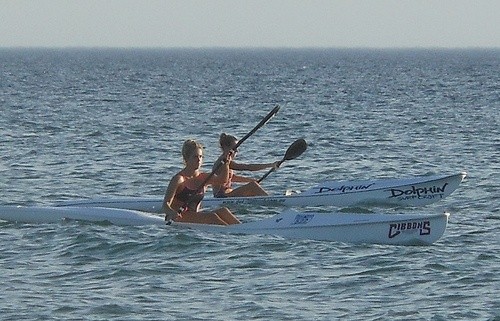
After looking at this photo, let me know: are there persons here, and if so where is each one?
[162,139,243,226]
[212,132,280,198]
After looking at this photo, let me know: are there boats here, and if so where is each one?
[0,204,451,247]
[52,171,468,213]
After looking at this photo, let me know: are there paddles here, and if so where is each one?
[257,139,308,183]
[165,105,281,225]
